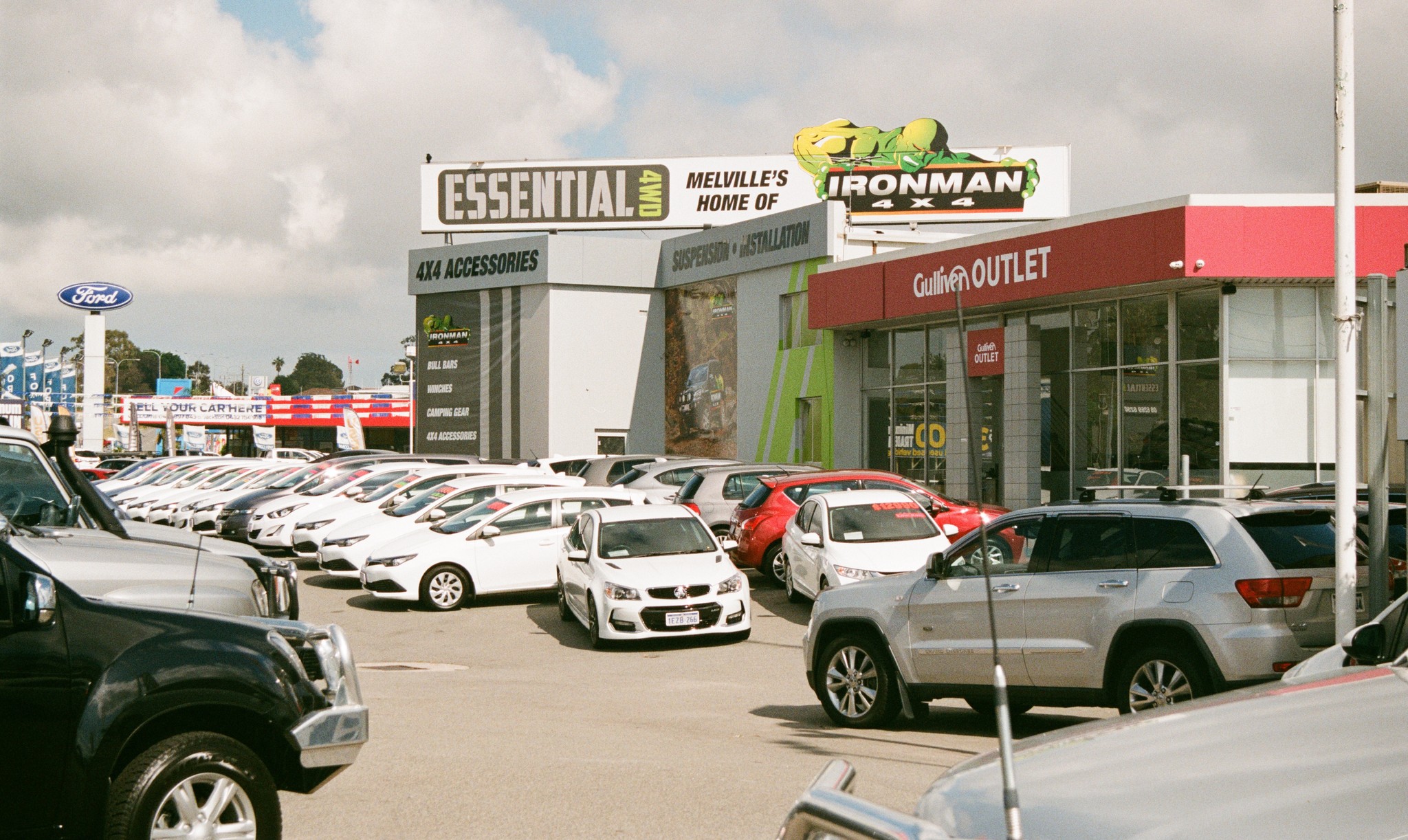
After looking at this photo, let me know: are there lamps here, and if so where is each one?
[703,223,712,231]
[549,228,558,235]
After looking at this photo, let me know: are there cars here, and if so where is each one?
[1280,592,1408,681]
[556,504,751,648]
[317,471,586,578]
[359,484,653,611]
[782,481,966,603]
[906,450,1221,511]
[50,448,480,544]
[290,462,544,558]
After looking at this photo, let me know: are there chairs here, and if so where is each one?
[536,502,565,525]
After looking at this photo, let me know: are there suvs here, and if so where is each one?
[1292,499,1407,601]
[0,511,367,840]
[677,359,725,436]
[1263,479,1407,503]
[672,464,827,567]
[575,456,700,488]
[803,473,1396,729]
[725,465,1025,589]
[772,277,1408,840]
[536,455,607,475]
[0,415,299,623]
[606,458,747,505]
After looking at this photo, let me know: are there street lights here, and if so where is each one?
[105,357,141,413]
[60,346,71,406]
[393,346,416,454]
[41,339,54,410]
[174,350,251,397]
[74,354,85,417]
[22,329,34,399]
[75,361,115,366]
[144,350,173,378]
